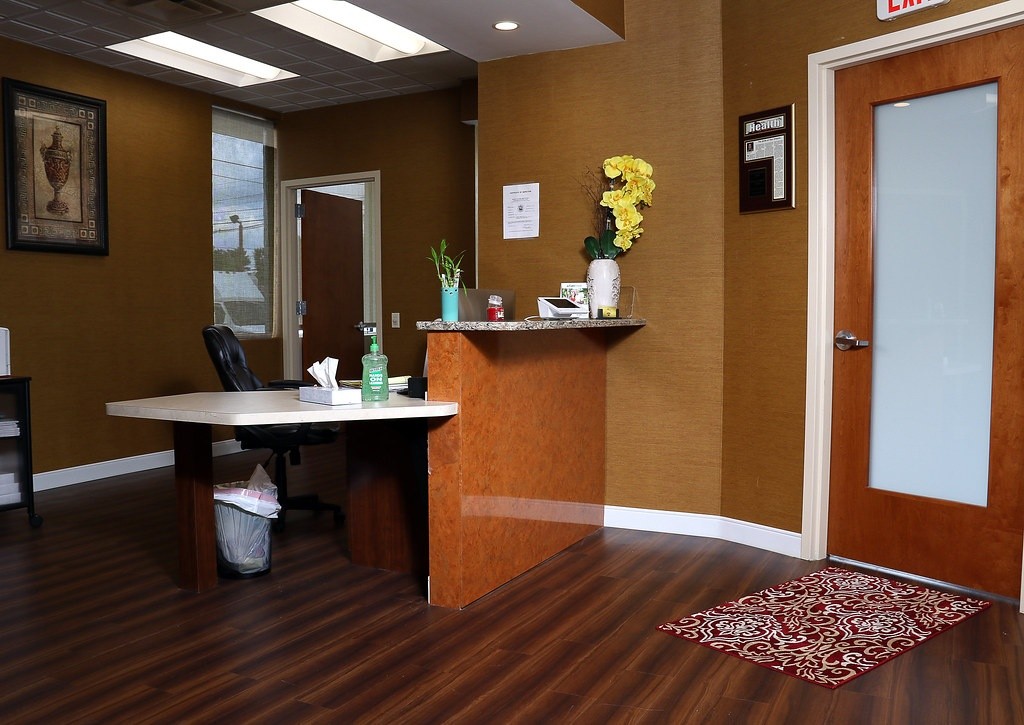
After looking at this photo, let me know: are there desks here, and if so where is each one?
[105,391,457,596]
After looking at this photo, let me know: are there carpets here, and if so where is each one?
[655,566,992,690]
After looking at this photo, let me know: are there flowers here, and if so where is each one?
[573,155,657,259]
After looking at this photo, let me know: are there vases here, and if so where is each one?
[586,259,621,319]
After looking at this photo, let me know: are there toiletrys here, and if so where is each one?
[362,336,389,401]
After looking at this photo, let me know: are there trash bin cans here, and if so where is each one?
[213,477,282,576]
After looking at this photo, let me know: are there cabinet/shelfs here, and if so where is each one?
[0,374,43,528]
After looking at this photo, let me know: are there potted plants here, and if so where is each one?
[426,240,467,322]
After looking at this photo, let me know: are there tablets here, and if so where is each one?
[538,297,589,314]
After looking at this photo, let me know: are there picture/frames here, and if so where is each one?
[0,77,109,257]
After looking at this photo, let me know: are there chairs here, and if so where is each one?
[201,323,345,535]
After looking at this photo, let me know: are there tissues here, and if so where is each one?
[299,358,361,405]
